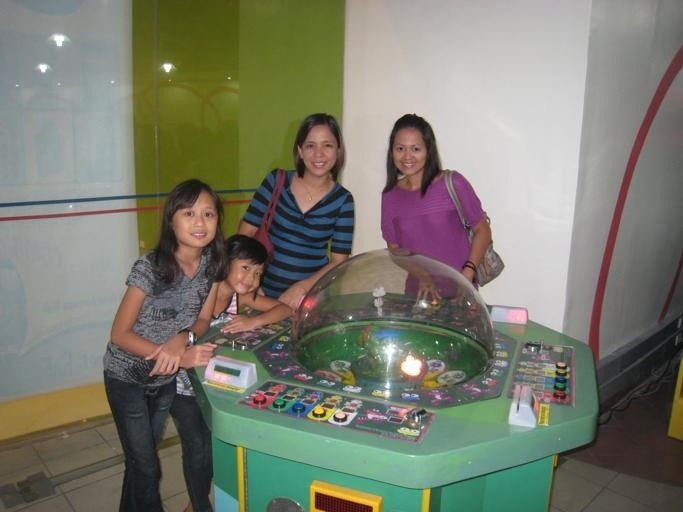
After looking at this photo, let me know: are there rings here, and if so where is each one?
[459,294,466,299]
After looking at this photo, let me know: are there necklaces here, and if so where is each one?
[300,176,329,201]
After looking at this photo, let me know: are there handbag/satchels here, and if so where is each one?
[256,223,273,265]
[465,227,504,287]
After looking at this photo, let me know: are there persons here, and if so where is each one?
[378,111,492,308]
[169,233,294,511]
[100,176,230,511]
[235,112,356,315]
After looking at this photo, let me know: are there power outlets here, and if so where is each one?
[675,318,682,347]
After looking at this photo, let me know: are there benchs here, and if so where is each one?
[0,382,114,448]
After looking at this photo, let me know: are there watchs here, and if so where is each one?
[176,327,197,350]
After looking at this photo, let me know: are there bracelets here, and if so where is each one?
[463,260,476,269]
[462,264,478,274]
